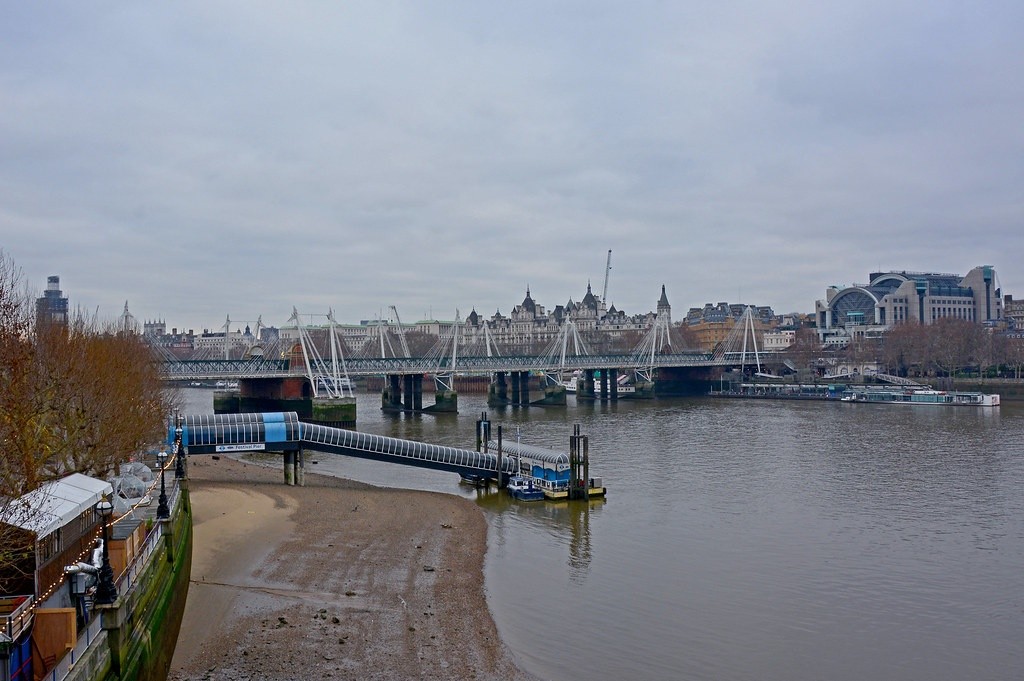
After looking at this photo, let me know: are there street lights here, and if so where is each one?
[157,445,170,518]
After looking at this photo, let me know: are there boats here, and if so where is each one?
[216,381,238,388]
[708,374,829,400]
[840,383,1001,407]
[481,425,572,502]
[558,370,635,395]
